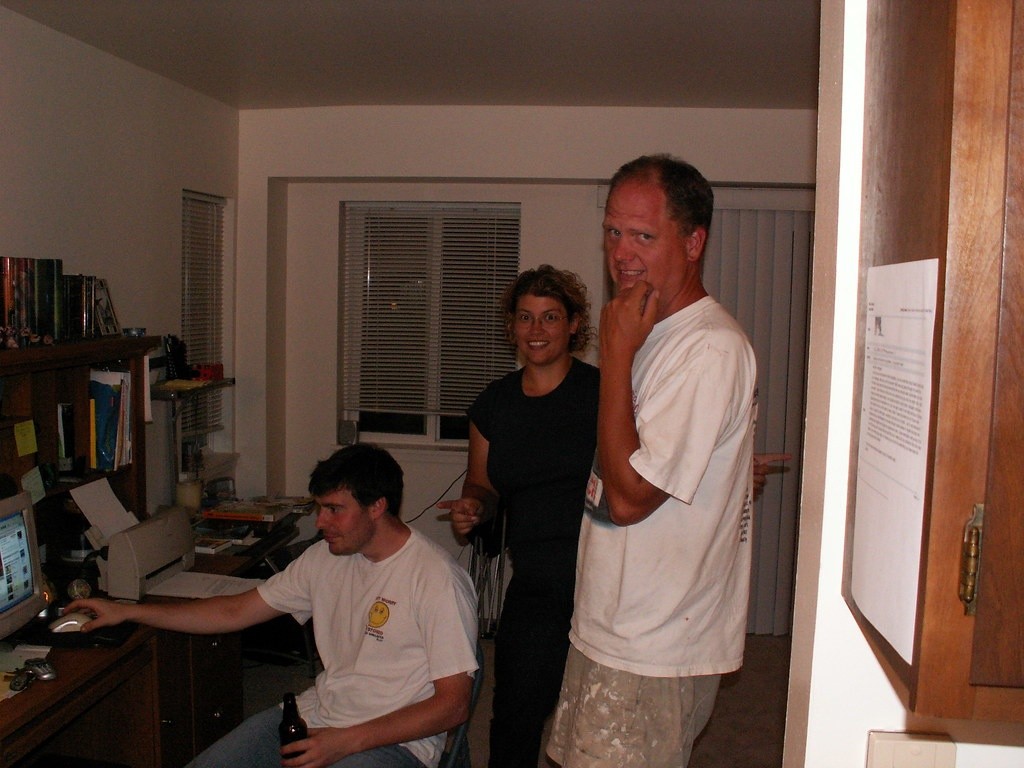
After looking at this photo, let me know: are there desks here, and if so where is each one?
[160,491,318,768]
[1,597,167,768]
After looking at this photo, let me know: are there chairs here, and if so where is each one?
[439,638,484,768]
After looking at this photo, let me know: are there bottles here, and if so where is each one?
[279,692,307,760]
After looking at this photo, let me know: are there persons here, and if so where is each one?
[547,155,756,768]
[63,444,479,768]
[437,265,792,768]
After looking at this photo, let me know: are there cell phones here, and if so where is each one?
[25,658,57,681]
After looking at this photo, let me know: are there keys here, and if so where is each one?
[3,663,37,692]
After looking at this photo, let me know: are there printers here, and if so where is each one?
[83,503,195,601]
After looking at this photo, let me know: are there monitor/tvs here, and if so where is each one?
[0,490,44,640]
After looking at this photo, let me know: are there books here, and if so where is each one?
[90,371,132,473]
[0,257,133,350]
[195,497,315,555]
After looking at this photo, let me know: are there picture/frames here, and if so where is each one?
[91,279,121,336]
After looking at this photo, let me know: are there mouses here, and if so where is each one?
[48,612,92,632]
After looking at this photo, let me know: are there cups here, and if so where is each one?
[176,484,201,516]
[61,559,98,602]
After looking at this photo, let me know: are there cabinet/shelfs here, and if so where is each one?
[158,376,238,488]
[1,337,161,596]
